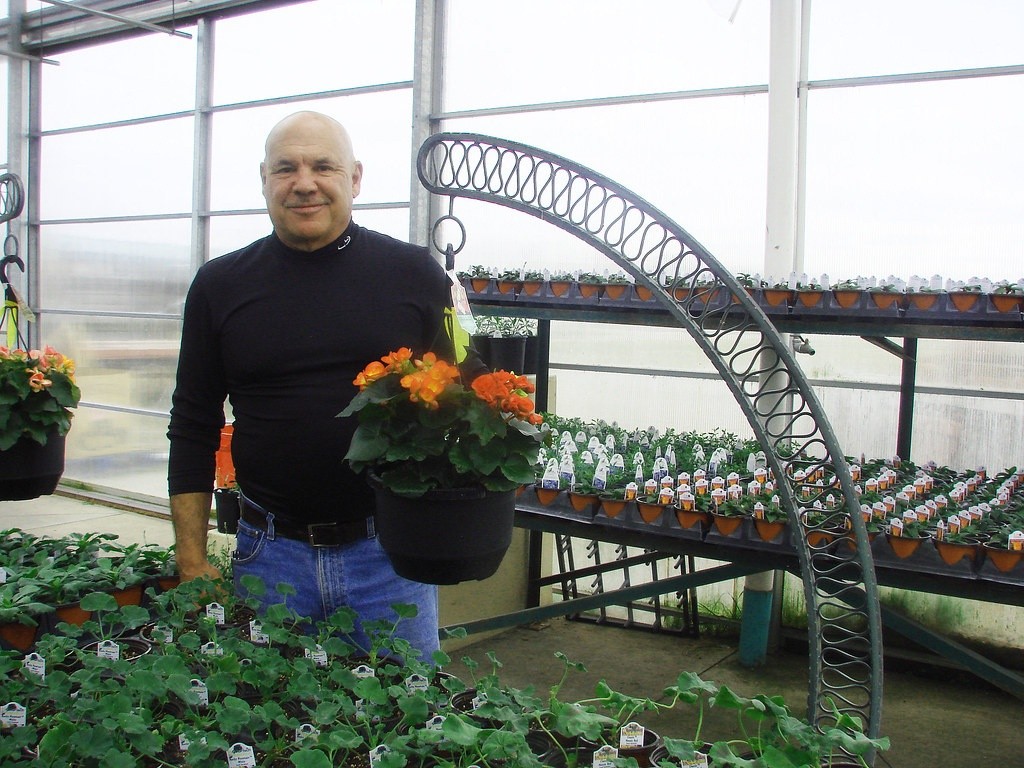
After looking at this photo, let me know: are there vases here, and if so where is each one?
[0,434,64,501]
[372,479,515,587]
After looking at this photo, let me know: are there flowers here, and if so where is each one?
[0,342,81,451]
[333,345,555,500]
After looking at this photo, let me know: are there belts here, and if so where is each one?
[237,505,377,548]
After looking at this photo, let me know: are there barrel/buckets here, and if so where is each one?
[215,425,236,489]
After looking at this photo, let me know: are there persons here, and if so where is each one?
[166,110,491,673]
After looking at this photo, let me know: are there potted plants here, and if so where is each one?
[0,261,1024,768]
